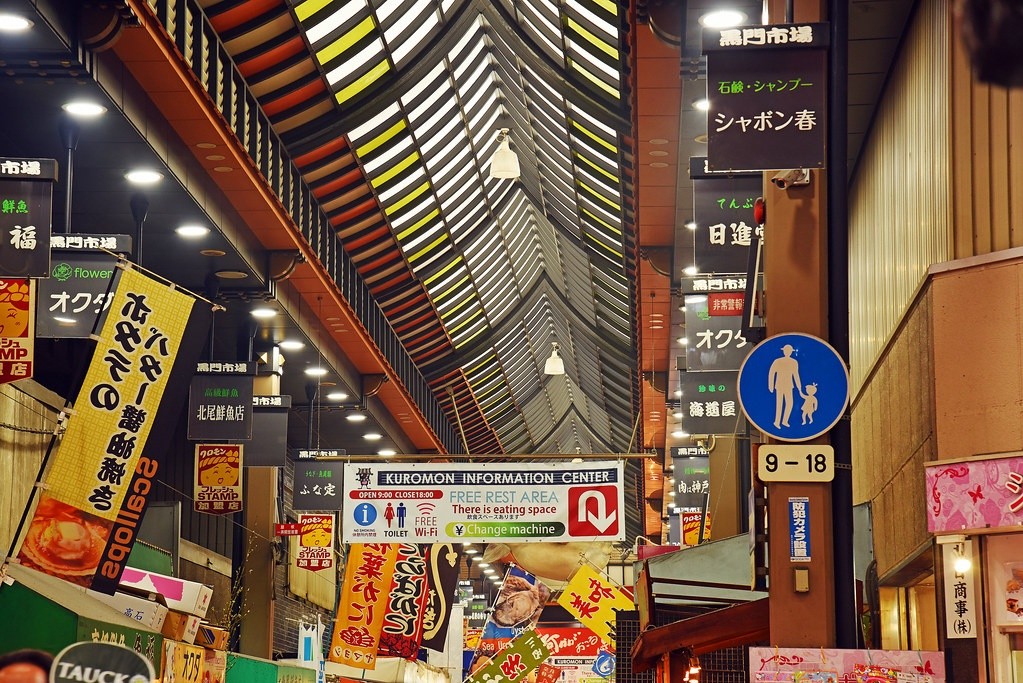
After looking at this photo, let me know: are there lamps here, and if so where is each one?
[544,342,564,375]
[489,128,521,179]
[571,448,582,462]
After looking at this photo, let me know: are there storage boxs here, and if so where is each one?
[84,564,229,650]
